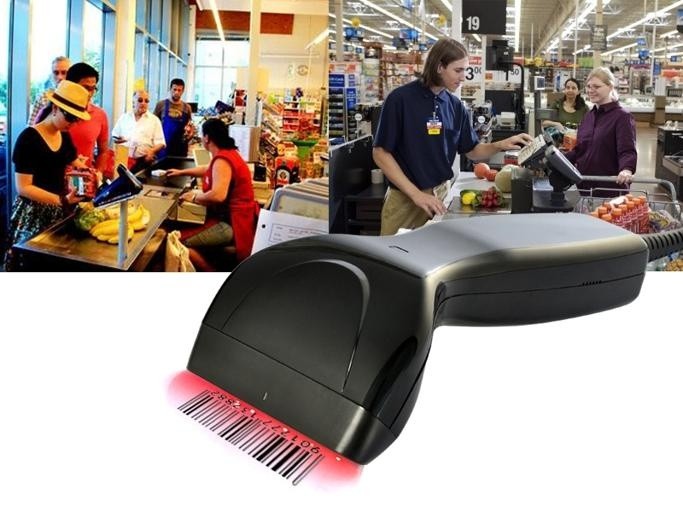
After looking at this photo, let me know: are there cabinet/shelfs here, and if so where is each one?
[580,96,683,122]
[260,94,329,178]
[329,38,418,144]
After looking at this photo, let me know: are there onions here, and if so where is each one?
[473,162,490,179]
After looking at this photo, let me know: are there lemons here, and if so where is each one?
[461,191,476,205]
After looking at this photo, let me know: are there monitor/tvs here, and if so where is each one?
[486,46,514,73]
[540,141,584,194]
[194,147,211,187]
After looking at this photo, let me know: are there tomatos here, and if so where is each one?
[487,170,498,182]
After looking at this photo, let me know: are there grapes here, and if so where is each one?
[481,186,501,207]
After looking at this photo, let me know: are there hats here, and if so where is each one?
[46,80,91,121]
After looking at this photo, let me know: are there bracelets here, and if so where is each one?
[59,193,71,208]
[192,195,197,203]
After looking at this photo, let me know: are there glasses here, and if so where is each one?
[137,98,150,103]
[59,108,79,123]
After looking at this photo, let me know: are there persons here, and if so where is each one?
[562,66,638,199]
[1,78,97,272]
[541,76,589,135]
[111,89,167,170]
[370,37,535,236]
[33,61,110,193]
[152,76,195,159]
[25,55,71,125]
[163,117,256,270]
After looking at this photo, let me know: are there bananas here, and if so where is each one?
[103,198,151,230]
[89,218,134,243]
